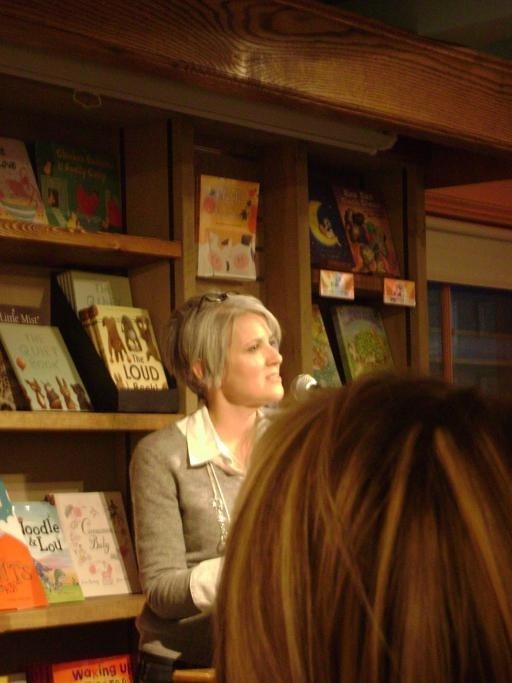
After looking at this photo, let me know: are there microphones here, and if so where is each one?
[292,373,319,403]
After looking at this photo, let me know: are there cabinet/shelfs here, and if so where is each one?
[1,46,429,633]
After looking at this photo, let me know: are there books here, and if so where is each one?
[0,137,125,237]
[0,262,169,412]
[309,181,403,279]
[0,478,147,613]
[1,652,158,683]
[311,301,399,383]
[195,173,261,283]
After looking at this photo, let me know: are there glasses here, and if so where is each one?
[192,290,241,313]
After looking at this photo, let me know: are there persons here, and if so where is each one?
[211,366,511,683]
[128,291,286,683]
